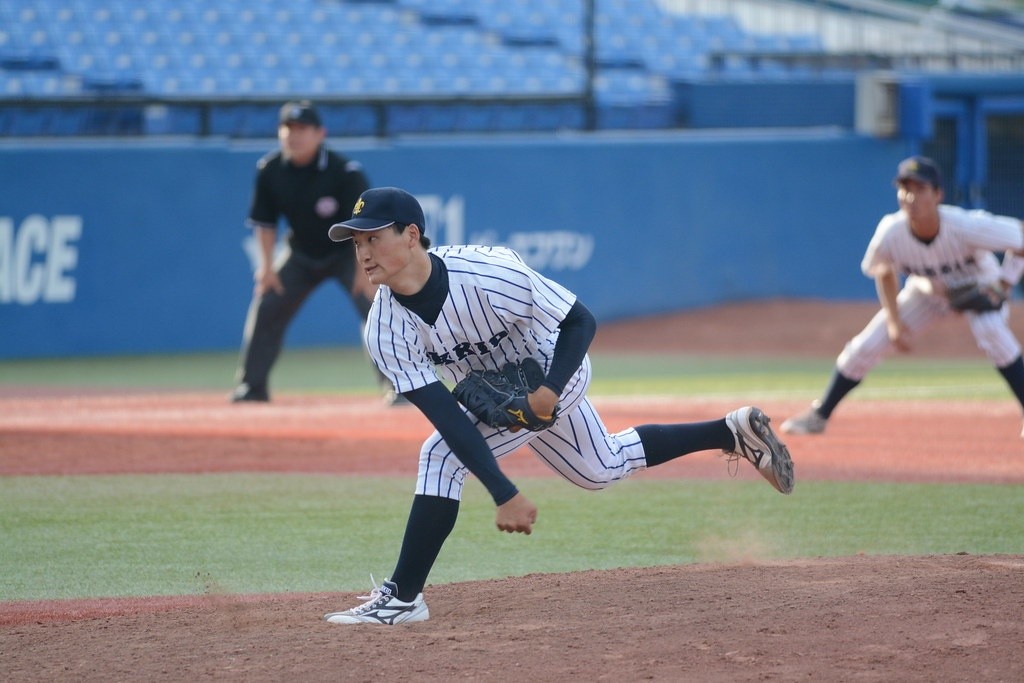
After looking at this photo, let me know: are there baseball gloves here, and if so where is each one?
[949,284,1004,315]
[450,356,561,434]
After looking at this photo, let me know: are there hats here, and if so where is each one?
[329,187,425,242]
[896,156,948,197]
[278,102,319,128]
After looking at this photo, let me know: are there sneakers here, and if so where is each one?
[323,574,430,625]
[721,405,795,495]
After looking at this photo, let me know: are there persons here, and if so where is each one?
[780,155,1024,438]
[322,186,795,626]
[231,100,411,405]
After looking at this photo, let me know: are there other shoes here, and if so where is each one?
[233,388,268,403]
[779,414,824,436]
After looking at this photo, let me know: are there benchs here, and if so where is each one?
[0,0,894,138]
